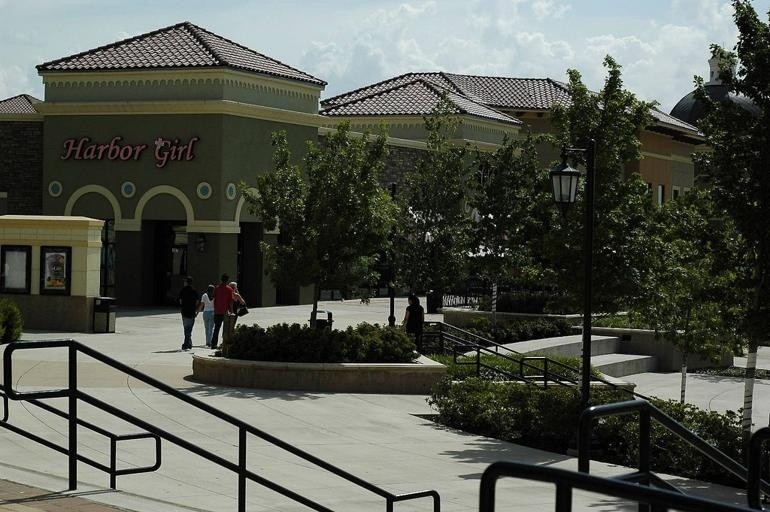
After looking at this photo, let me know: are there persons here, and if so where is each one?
[402,294,424,352]
[178,272,247,352]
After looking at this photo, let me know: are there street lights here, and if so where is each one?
[548,136,603,471]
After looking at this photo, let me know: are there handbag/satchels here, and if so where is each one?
[234,301,248,316]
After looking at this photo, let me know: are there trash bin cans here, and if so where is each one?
[93,297,117,333]
[308,310,334,331]
